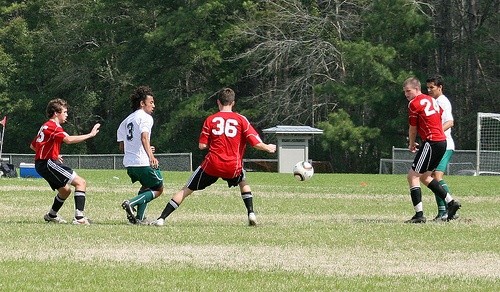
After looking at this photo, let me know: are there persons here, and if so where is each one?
[146,87,277,226]
[425,74,461,223]
[117,86,164,226]
[30,98,101,225]
[403,77,462,224]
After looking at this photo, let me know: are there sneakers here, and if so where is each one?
[431,212,459,221]
[44,212,68,224]
[447,202,461,222]
[155,217,165,226]
[404,215,426,223]
[248,213,257,225]
[70,217,91,225]
[121,201,137,224]
[135,216,157,226]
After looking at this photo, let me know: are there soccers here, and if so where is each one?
[293,161,314,181]
